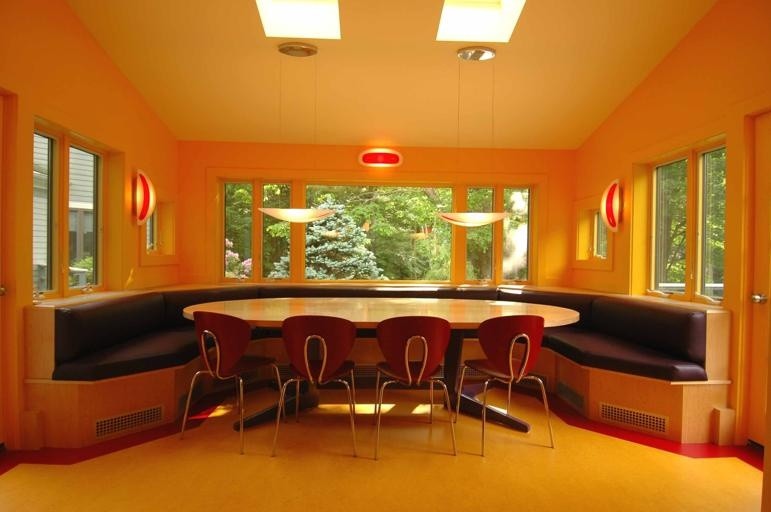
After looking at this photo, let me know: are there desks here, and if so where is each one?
[181,295,583,432]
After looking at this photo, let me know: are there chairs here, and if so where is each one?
[269,315,358,458]
[375,315,457,460]
[455,315,556,456]
[178,309,288,455]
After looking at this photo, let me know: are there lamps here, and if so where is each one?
[437,45,512,229]
[357,148,403,167]
[598,178,623,232]
[131,167,156,227]
[255,42,335,225]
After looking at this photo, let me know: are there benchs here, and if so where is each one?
[19,280,731,450]
